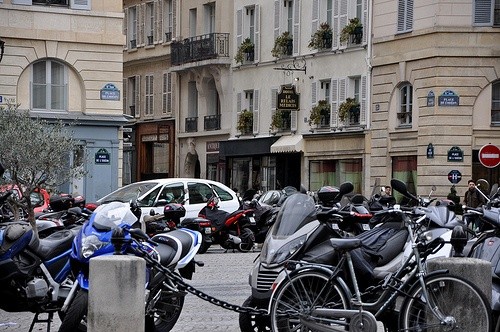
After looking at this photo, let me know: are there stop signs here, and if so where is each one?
[478,144,500,169]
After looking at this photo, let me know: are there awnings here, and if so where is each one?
[270,135,304,154]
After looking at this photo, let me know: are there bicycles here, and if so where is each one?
[266,205,494,331]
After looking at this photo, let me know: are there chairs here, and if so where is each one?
[206,193,221,201]
[179,193,204,204]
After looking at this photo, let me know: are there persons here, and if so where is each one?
[463,180,485,233]
[381,186,391,197]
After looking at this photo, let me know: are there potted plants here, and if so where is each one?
[234,38,254,64]
[340,18,362,44]
[238,109,253,132]
[271,32,293,58]
[308,23,333,49]
[271,108,290,130]
[309,100,330,126]
[338,98,361,122]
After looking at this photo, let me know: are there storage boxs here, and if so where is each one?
[74,195,85,207]
[49,191,74,209]
[318,186,342,204]
[164,203,186,218]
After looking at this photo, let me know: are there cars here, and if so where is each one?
[97,176,241,225]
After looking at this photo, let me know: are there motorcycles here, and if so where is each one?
[0,177,499,332]
